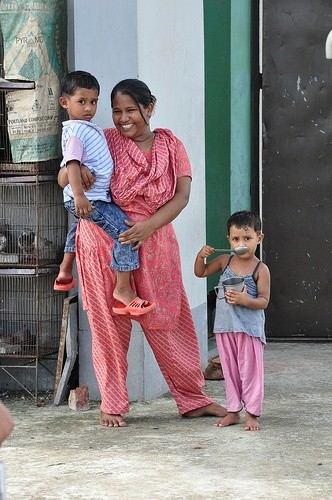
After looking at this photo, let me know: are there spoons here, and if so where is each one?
[213,247,249,254]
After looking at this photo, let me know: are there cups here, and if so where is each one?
[213,277,245,303]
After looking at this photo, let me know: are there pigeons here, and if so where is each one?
[18,228,53,258]
[0,224,14,255]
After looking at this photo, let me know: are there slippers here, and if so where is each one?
[112,297,155,315]
[54,276,77,291]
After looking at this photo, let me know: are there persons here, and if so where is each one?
[0,401,14,447]
[58,77,228,427]
[53,71,156,317]
[194,210,271,430]
